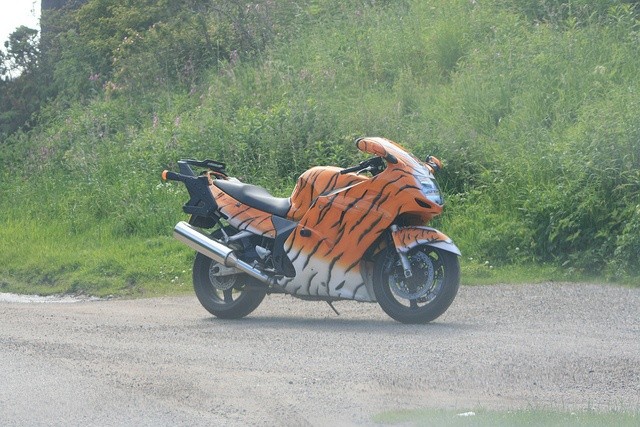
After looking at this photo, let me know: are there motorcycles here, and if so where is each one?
[162,137,462,324]
